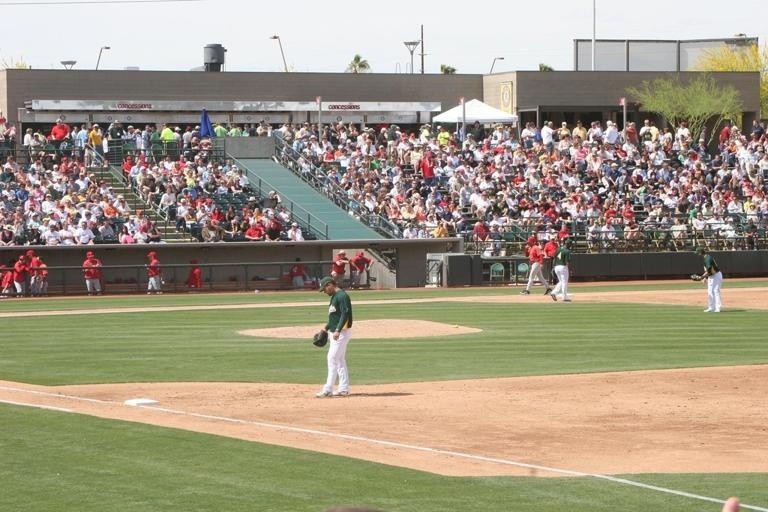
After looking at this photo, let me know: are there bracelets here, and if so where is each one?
[335,329,340,332]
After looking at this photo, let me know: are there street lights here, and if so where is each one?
[488,57,505,76]
[269,36,288,72]
[61,61,76,71]
[96,46,112,71]
[404,41,419,73]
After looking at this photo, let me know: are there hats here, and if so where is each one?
[26,250,35,256]
[550,235,556,239]
[319,277,334,291]
[695,246,704,255]
[337,249,347,255]
[527,237,536,243]
[147,251,157,257]
[563,238,574,245]
[20,255,26,260]
[357,251,364,257]
[86,251,95,258]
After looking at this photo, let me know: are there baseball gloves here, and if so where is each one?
[691,274,704,281]
[313,330,327,346]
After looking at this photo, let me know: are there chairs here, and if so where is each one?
[0,139,322,246]
[273,122,768,246]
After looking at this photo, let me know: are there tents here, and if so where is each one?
[431,98,522,141]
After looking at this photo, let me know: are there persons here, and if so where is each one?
[82,250,103,296]
[722,497,740,511]
[690,247,723,313]
[520,236,573,303]
[145,251,163,295]
[2,249,48,298]
[314,276,353,398]
[0,116,305,246]
[185,259,201,289]
[277,114,768,256]
[329,251,373,288]
[290,257,307,287]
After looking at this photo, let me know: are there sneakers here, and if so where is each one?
[315,391,334,398]
[562,299,571,303]
[520,289,529,295]
[550,293,557,301]
[544,288,551,295]
[335,390,350,398]
